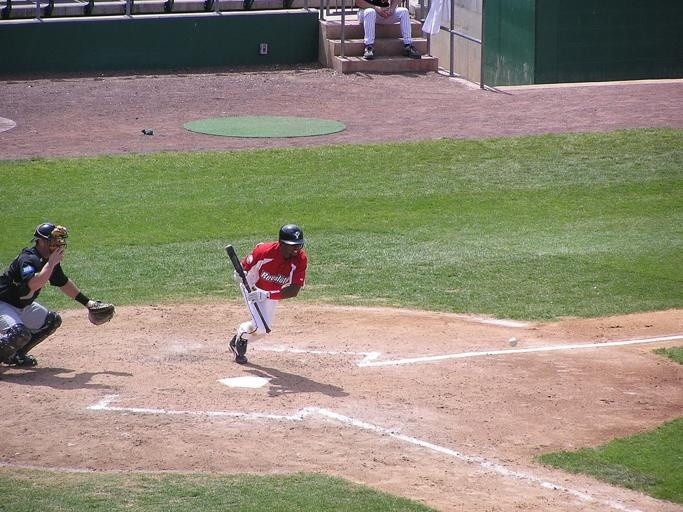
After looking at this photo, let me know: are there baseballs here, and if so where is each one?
[508,338,517,347]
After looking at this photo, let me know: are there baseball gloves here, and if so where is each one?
[89,301,114,326]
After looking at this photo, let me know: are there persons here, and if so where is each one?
[0,223,113,368]
[356,1,423,59]
[226,223,307,365]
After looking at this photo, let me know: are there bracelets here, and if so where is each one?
[268,290,283,300]
[74,291,91,306]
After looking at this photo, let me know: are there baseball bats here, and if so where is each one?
[224,245,271,333]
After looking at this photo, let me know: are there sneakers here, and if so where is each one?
[228,335,248,364]
[0,353,36,368]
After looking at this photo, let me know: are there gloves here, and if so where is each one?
[248,284,268,303]
[233,269,247,282]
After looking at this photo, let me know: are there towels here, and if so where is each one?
[421,0,452,35]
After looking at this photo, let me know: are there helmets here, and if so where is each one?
[34,223,67,253]
[279,224,304,245]
